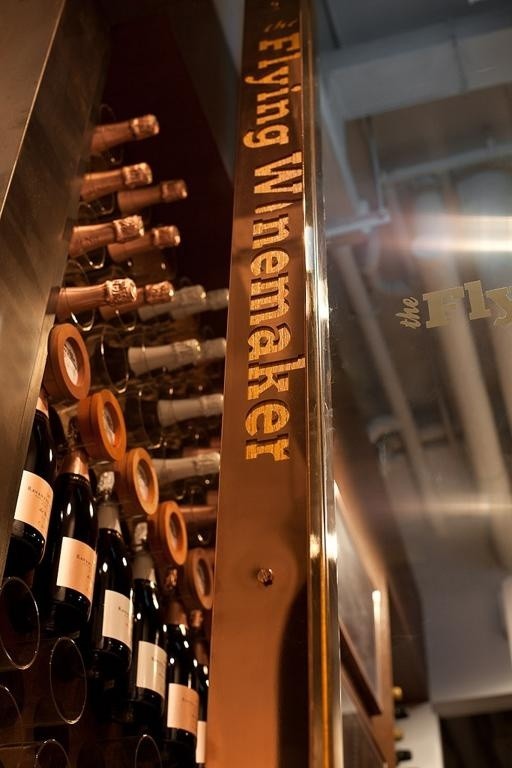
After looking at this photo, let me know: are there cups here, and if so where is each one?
[1,575,163,767]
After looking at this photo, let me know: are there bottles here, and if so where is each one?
[7,372,210,768]
[49,107,229,528]
